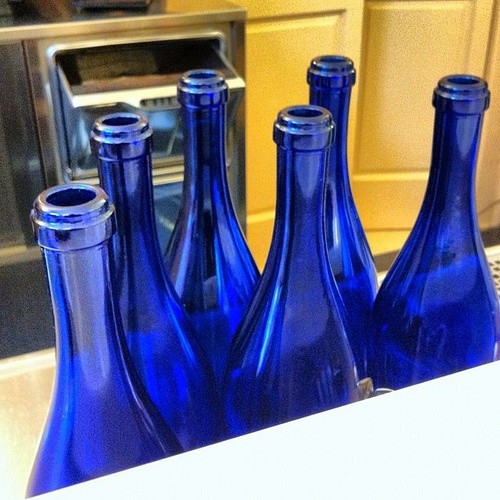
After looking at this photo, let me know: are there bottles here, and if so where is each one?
[24,55,500,500]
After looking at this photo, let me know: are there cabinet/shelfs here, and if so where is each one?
[162,0,500,276]
